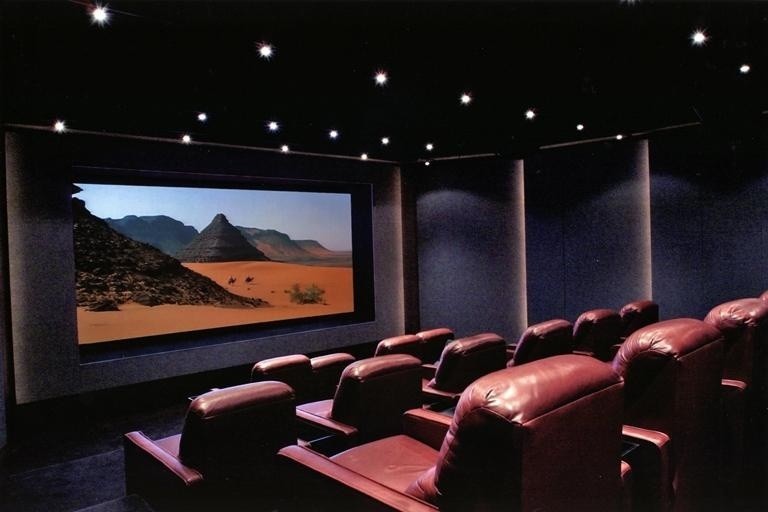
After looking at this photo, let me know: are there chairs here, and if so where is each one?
[278,354,630,512]
[375,328,456,364]
[422,332,508,412]
[575,309,620,359]
[124,380,333,511]
[250,353,356,405]
[605,294,768,510]
[508,319,576,366]
